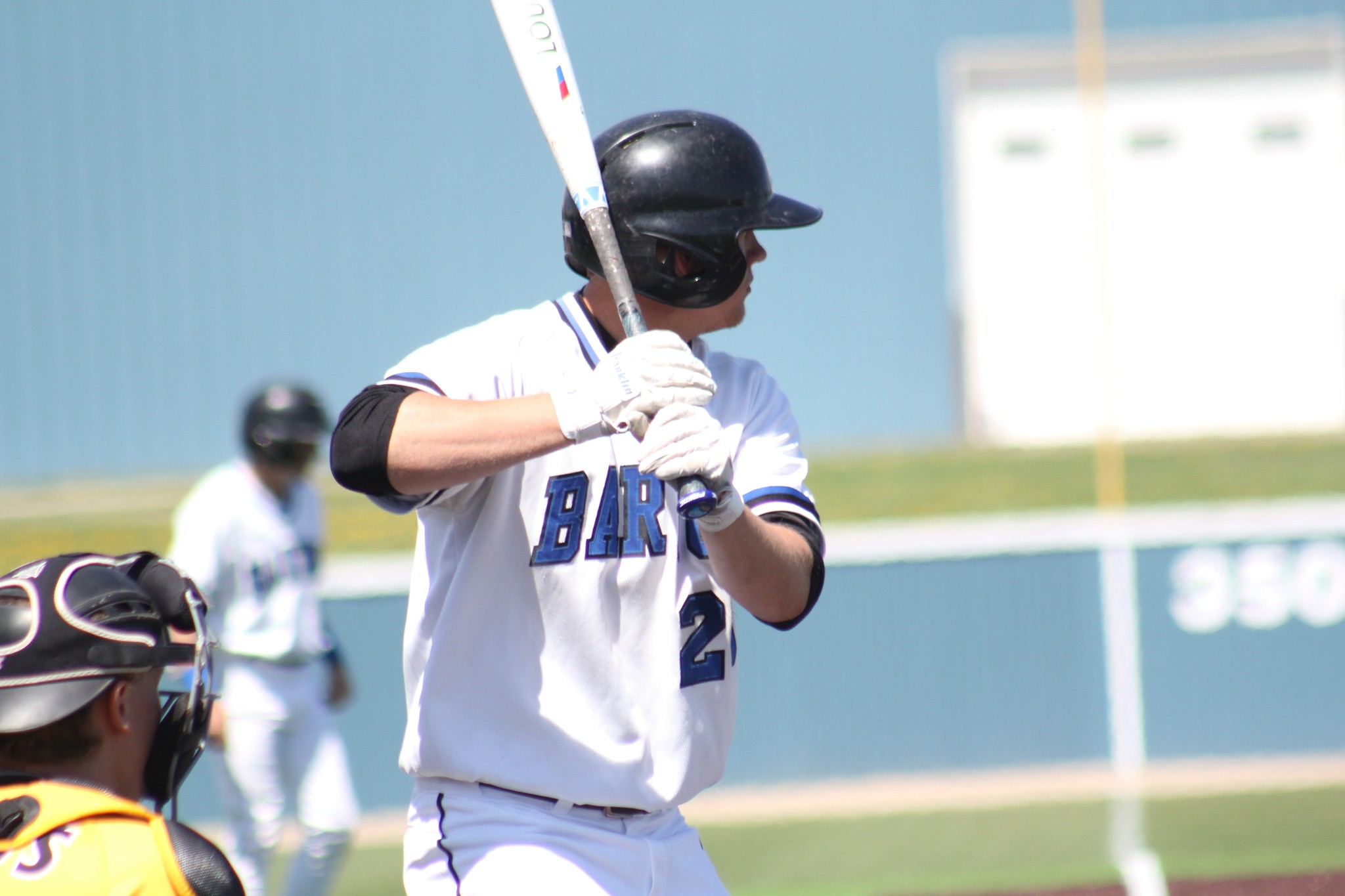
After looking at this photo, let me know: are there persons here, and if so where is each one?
[328,111,826,896]
[0,551,246,896]
[165,383,354,896]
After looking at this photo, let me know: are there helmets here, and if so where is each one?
[561,110,822,310]
[1,552,166,737]
[240,383,326,468]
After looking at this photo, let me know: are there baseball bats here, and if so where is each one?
[489,0,718,521]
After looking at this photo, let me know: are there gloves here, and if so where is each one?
[627,402,744,533]
[551,329,716,445]
